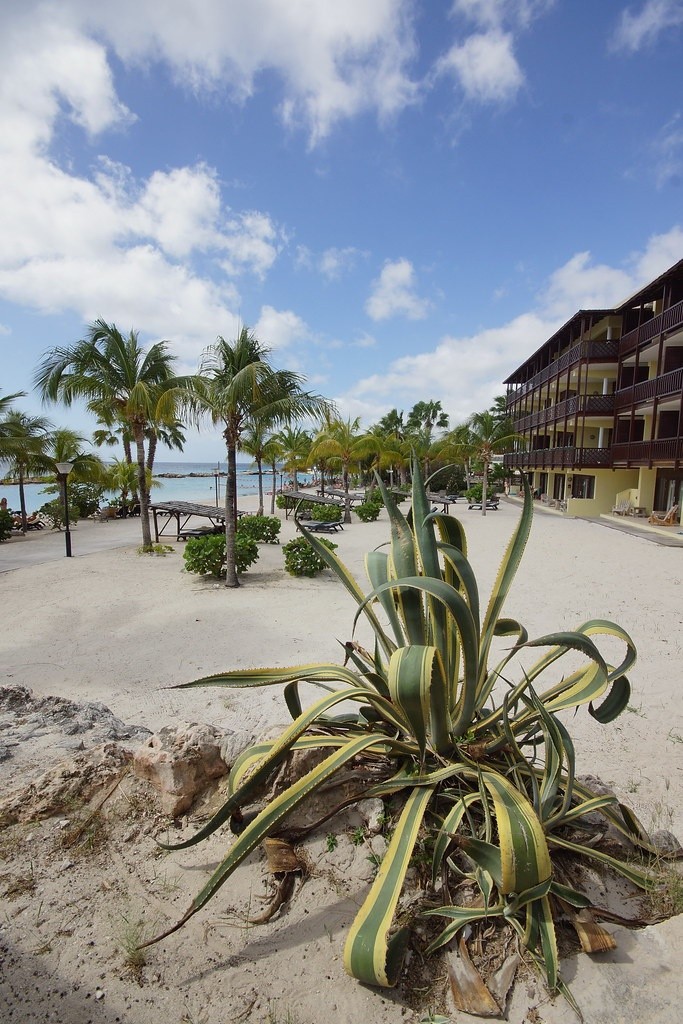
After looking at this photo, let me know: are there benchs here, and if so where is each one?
[612,499,631,517]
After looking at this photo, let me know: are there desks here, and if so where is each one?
[630,507,645,518]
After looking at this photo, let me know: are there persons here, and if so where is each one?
[282,474,354,492]
[504,478,510,497]
[0,498,7,511]
[10,512,37,523]
[519,485,537,499]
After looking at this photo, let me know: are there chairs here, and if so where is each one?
[116,506,129,518]
[307,521,345,529]
[541,494,554,504]
[17,515,46,532]
[468,501,500,510]
[177,525,219,539]
[648,505,679,526]
[92,508,119,523]
[128,504,141,517]
[296,523,338,535]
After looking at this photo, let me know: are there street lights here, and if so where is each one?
[212,467,219,522]
[54,462,74,558]
[278,470,285,494]
